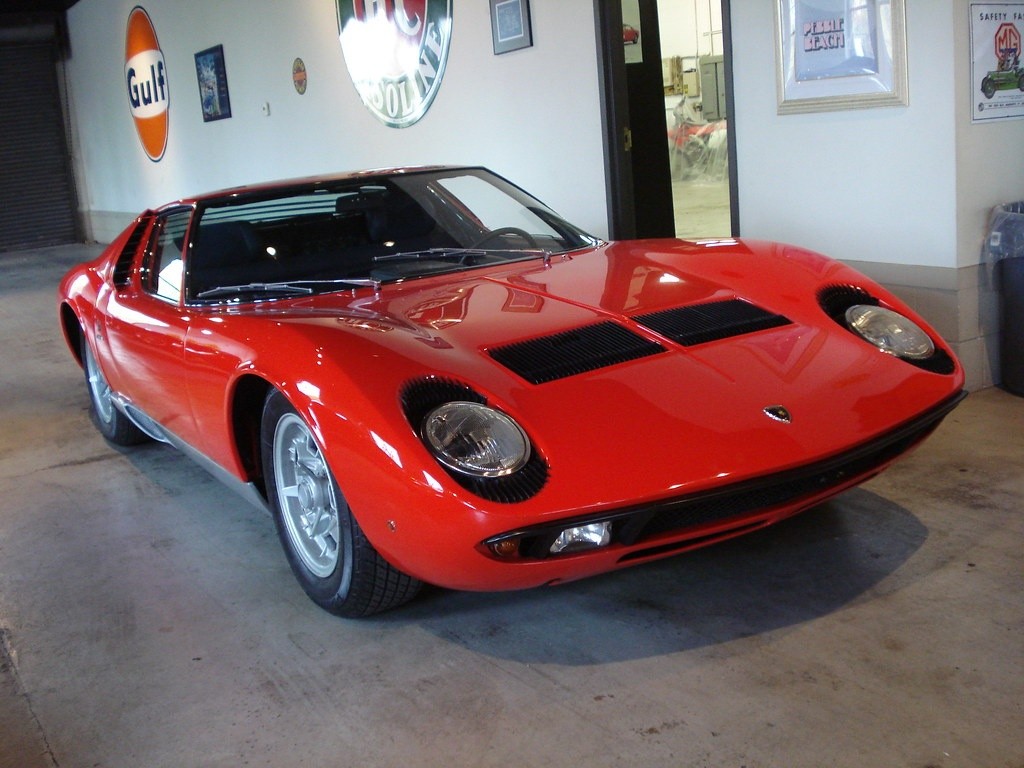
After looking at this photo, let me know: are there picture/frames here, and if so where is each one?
[489,0,533,56]
[772,0,910,117]
[193,44,233,124]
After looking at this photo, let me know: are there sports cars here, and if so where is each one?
[56,166,967,620]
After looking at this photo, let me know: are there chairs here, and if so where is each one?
[184,219,267,302]
[361,191,442,268]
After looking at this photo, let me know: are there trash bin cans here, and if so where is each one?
[993,200,1024,397]
